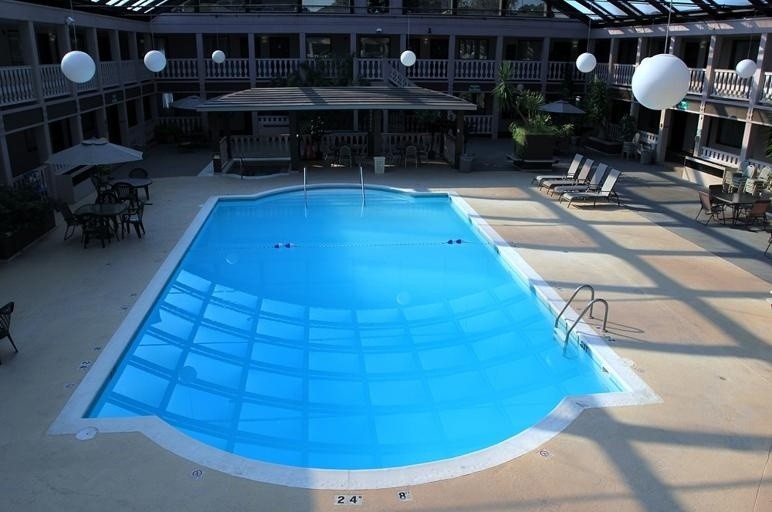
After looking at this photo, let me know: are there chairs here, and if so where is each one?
[339,146,352,168]
[56,167,149,250]
[404,146,418,168]
[1,301,18,364]
[694,161,772,232]
[531,153,621,208]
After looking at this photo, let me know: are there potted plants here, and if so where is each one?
[488,60,568,171]
[620,114,638,152]
[303,115,332,160]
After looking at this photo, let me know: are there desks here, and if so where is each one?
[109,178,153,200]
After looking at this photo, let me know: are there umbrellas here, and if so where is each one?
[44,139,143,204]
[171,95,207,132]
[537,100,586,125]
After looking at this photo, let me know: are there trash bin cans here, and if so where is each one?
[373,156,386,174]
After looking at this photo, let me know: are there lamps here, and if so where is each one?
[736,14,757,79]
[575,1,597,73]
[60,1,97,83]
[144,7,167,73]
[630,0,691,111]
[212,15,226,65]
[400,10,417,67]
